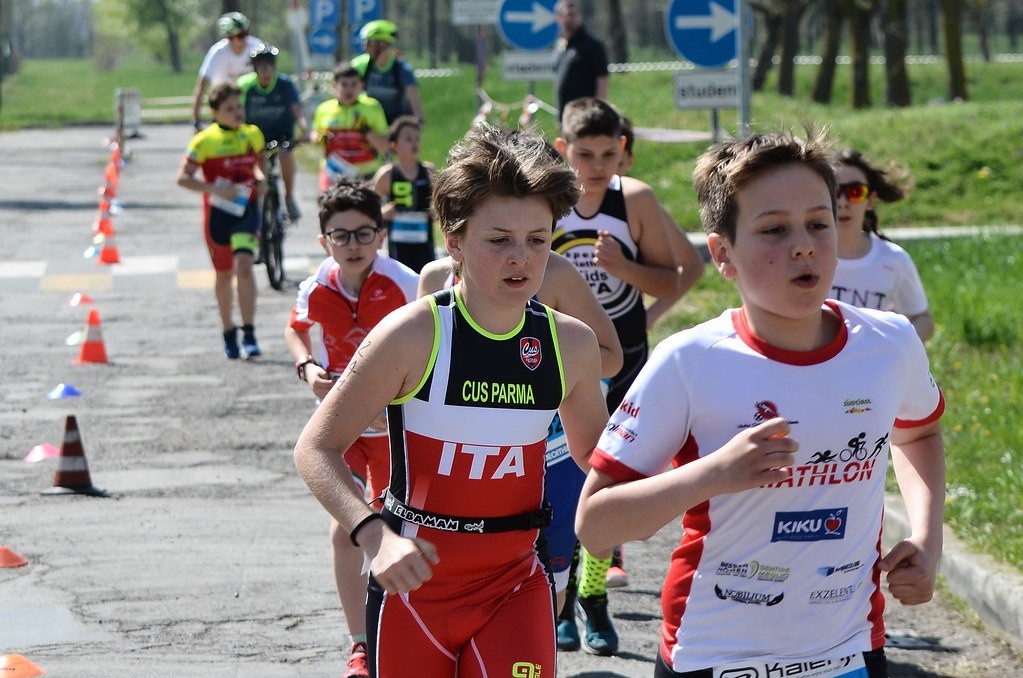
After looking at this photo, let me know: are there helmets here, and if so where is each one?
[215,11,250,38]
[359,19,398,43]
[248,41,280,59]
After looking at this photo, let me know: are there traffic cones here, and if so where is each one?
[38,415,108,495]
[98,223,120,264]
[70,309,109,363]
[96,199,116,238]
[100,148,122,200]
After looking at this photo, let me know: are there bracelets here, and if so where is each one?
[192,119,199,128]
[350,512,386,548]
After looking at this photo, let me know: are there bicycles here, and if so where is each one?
[264,139,310,291]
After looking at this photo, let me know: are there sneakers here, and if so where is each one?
[344,639,370,678]
[575,593,618,655]
[556,585,580,651]
[242,336,261,361]
[604,550,628,587]
[223,336,239,359]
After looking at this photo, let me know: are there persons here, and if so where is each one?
[348,19,425,127]
[234,43,311,264]
[550,0,611,133]
[282,175,421,678]
[176,81,273,362]
[826,145,935,342]
[573,111,947,678]
[293,122,610,678]
[369,113,437,274]
[308,63,392,192]
[415,96,707,657]
[191,11,269,137]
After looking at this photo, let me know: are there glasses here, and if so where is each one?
[324,225,381,248]
[835,182,870,202]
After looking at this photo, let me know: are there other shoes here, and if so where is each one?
[285,194,300,221]
[269,269,282,291]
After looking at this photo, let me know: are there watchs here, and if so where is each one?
[294,354,315,382]
